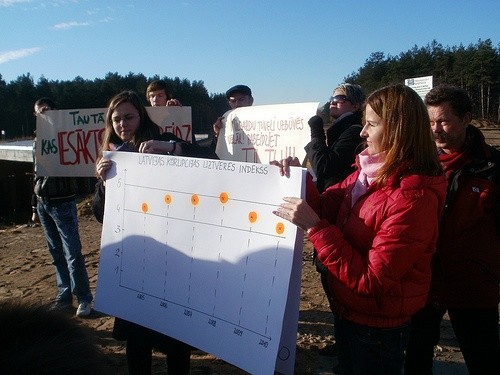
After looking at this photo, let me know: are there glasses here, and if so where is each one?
[111,115,135,123]
[329,94,353,103]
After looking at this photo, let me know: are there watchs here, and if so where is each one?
[167,139,177,155]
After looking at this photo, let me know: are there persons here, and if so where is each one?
[146,80,182,107]
[32,97,93,316]
[403,85,500,375]
[209,85,253,160]
[304,83,368,375]
[270,83,447,375]
[92,90,201,375]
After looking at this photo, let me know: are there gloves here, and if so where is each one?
[307,114,324,138]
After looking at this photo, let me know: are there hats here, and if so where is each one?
[225,84,253,97]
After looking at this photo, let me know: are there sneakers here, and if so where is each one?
[75,302,92,317]
[47,304,71,318]
